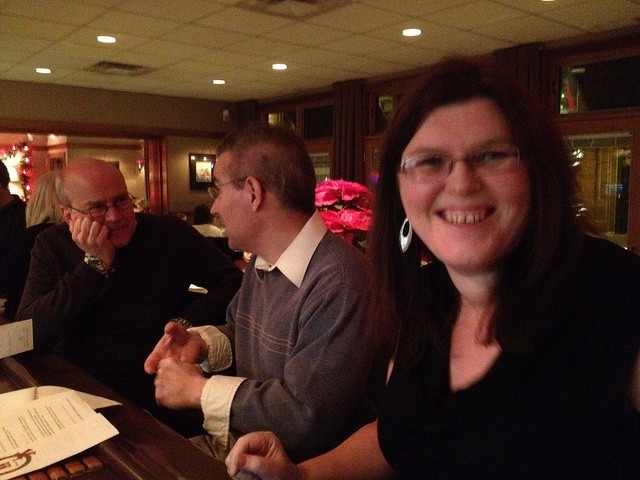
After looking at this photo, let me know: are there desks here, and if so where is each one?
[0,314,237,480]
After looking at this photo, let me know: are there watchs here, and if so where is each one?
[84,253,114,277]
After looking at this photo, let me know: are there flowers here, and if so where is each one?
[315,177,372,243]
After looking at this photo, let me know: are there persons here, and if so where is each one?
[0,161,65,321]
[226,64,640,480]
[16,158,244,435]
[143,126,393,466]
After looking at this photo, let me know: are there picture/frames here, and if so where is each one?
[188,153,216,191]
[47,152,65,172]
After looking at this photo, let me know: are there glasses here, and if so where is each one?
[398,144,523,184]
[208,176,248,200]
[65,192,136,218]
